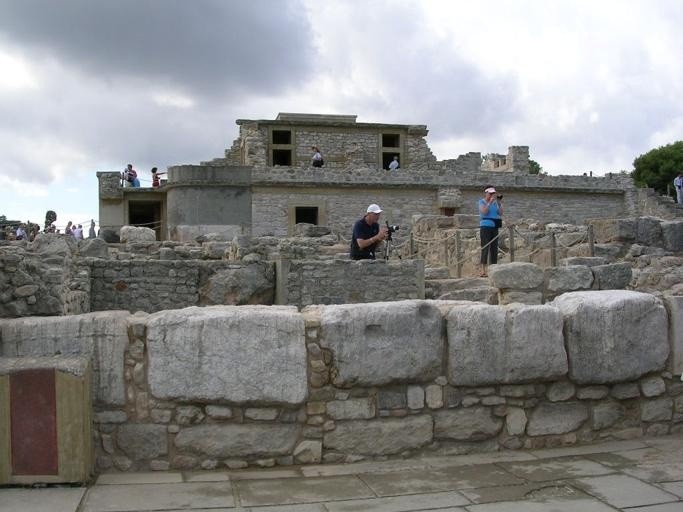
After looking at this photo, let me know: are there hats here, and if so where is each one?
[312,146,320,152]
[484,188,496,193]
[367,204,383,214]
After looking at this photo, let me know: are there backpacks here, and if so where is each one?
[313,158,324,167]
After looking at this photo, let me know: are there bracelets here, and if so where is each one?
[369,237,375,244]
[486,203,490,208]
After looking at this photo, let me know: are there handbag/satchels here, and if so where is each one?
[481,218,502,228]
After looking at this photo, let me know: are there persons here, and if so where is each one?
[388,156,399,171]
[150,167,164,187]
[673,173,682,205]
[309,146,322,167]
[4,221,82,241]
[477,185,504,278]
[349,202,392,261]
[122,164,137,187]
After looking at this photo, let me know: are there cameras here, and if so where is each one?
[497,194,503,200]
[386,220,399,232]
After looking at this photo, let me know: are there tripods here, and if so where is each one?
[384,231,403,261]
